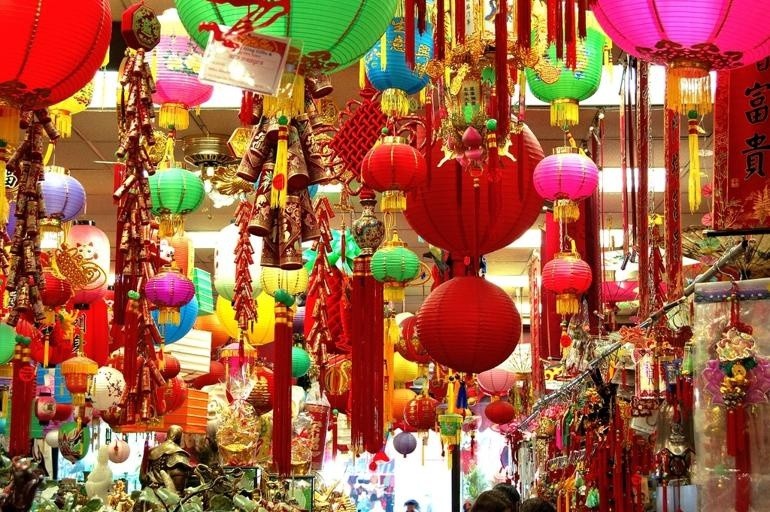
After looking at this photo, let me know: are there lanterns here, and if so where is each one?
[0,2,770,465]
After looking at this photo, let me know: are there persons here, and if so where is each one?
[517,496,557,511]
[270,480,299,505]
[139,423,192,494]
[467,488,512,511]
[493,481,522,507]
[401,498,421,512]
